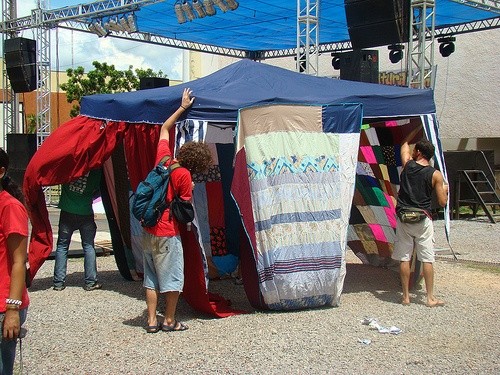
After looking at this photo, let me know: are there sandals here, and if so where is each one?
[147,322,163,332]
[163,320,188,332]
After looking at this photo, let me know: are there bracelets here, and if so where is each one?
[5,299,22,305]
[181,106,185,109]
[5,304,19,309]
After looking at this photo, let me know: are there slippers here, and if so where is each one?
[429,299,444,307]
[397,298,410,305]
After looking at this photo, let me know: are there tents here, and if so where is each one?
[23,58,457,316]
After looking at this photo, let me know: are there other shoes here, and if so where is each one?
[235,277,243,285]
[84,284,102,291]
[54,285,66,291]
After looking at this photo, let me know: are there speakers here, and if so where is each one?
[6,133,44,193]
[344,0,417,50]
[338,50,379,83]
[140,77,170,89]
[4,38,44,93]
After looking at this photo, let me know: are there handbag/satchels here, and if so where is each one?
[169,195,195,225]
[398,211,428,224]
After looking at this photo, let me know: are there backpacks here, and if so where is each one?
[130,156,182,228]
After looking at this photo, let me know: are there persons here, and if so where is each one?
[53,170,103,291]
[143,88,213,332]
[0,148,31,375]
[391,125,448,307]
